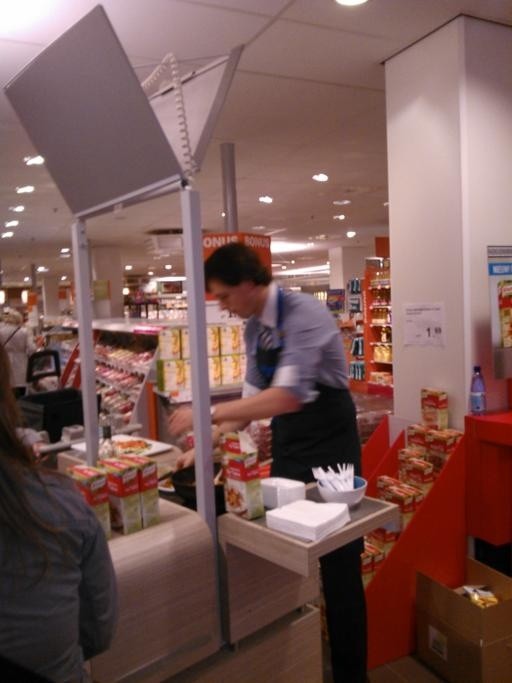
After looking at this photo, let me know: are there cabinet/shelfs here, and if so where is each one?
[38,317,81,391]
[155,325,249,447]
[362,269,394,400]
[314,291,367,392]
[46,318,162,439]
[358,424,465,669]
[356,412,390,484]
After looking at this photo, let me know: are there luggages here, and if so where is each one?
[14,349,102,445]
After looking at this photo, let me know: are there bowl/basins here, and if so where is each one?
[317,476,367,506]
[171,461,224,507]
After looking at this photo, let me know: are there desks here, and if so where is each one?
[58,435,398,682]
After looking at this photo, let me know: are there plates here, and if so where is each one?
[72,434,172,456]
[158,478,177,491]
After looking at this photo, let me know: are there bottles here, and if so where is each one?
[470,365,487,416]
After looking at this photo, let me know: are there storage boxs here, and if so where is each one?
[416,557,512,683]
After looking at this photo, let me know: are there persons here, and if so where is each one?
[1,302,11,321]
[0,340,116,683]
[203,241,370,683]
[17,303,29,325]
[0,310,36,399]
